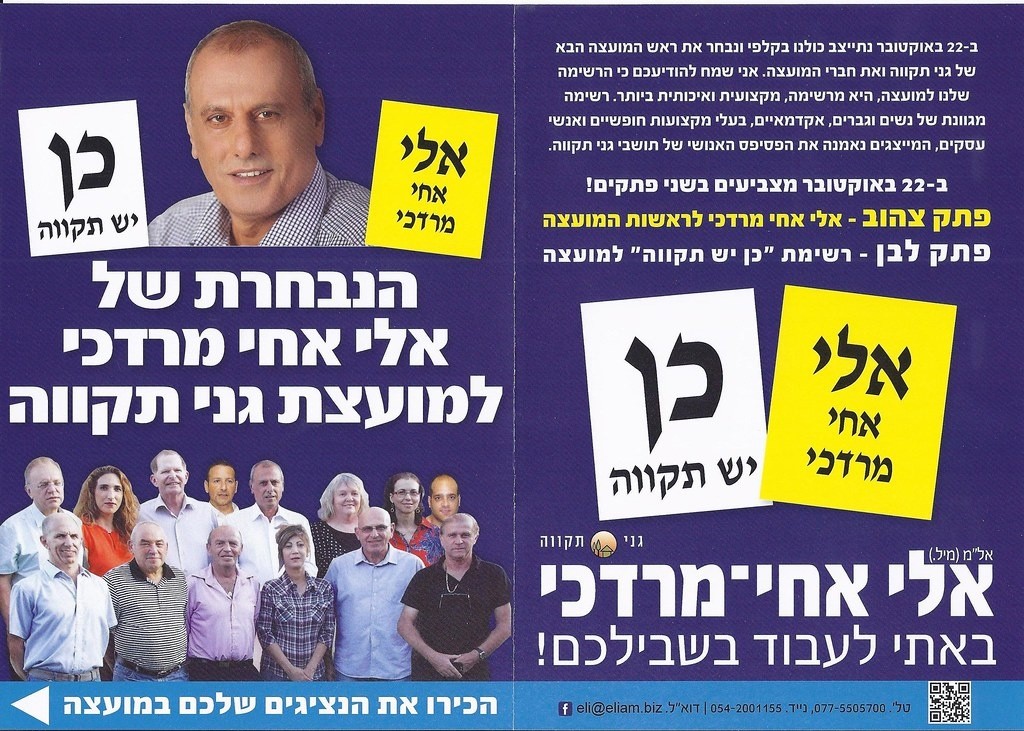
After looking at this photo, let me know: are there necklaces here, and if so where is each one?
[445,562,467,593]
[401,525,413,536]
[217,578,235,596]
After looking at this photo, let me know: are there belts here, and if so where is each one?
[205,659,253,667]
[116,654,186,679]
[29,668,100,682]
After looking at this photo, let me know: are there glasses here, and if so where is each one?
[439,594,472,613]
[392,489,422,497]
[27,480,62,489]
[359,525,391,533]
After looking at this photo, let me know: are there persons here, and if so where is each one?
[425,473,461,530]
[0,457,85,623]
[7,513,120,684]
[322,505,426,683]
[182,526,260,682]
[226,459,319,672]
[147,21,371,247]
[101,522,191,683]
[397,513,513,682]
[310,473,371,579]
[202,459,240,523]
[255,523,337,683]
[72,465,139,680]
[381,472,444,571]
[138,450,218,577]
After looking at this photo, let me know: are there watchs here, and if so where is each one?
[474,647,487,660]
